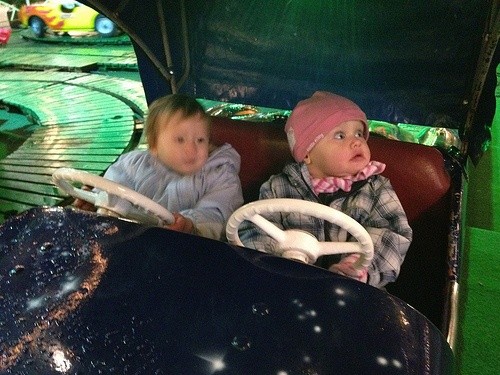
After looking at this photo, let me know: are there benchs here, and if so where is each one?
[210,114,453,326]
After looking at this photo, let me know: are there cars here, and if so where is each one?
[17,0,125,40]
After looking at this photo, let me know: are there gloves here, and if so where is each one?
[329,253,367,285]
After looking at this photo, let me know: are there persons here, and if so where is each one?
[71,94,243,239]
[238,90,412,288]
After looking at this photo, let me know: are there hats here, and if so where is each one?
[284,90,370,163]
